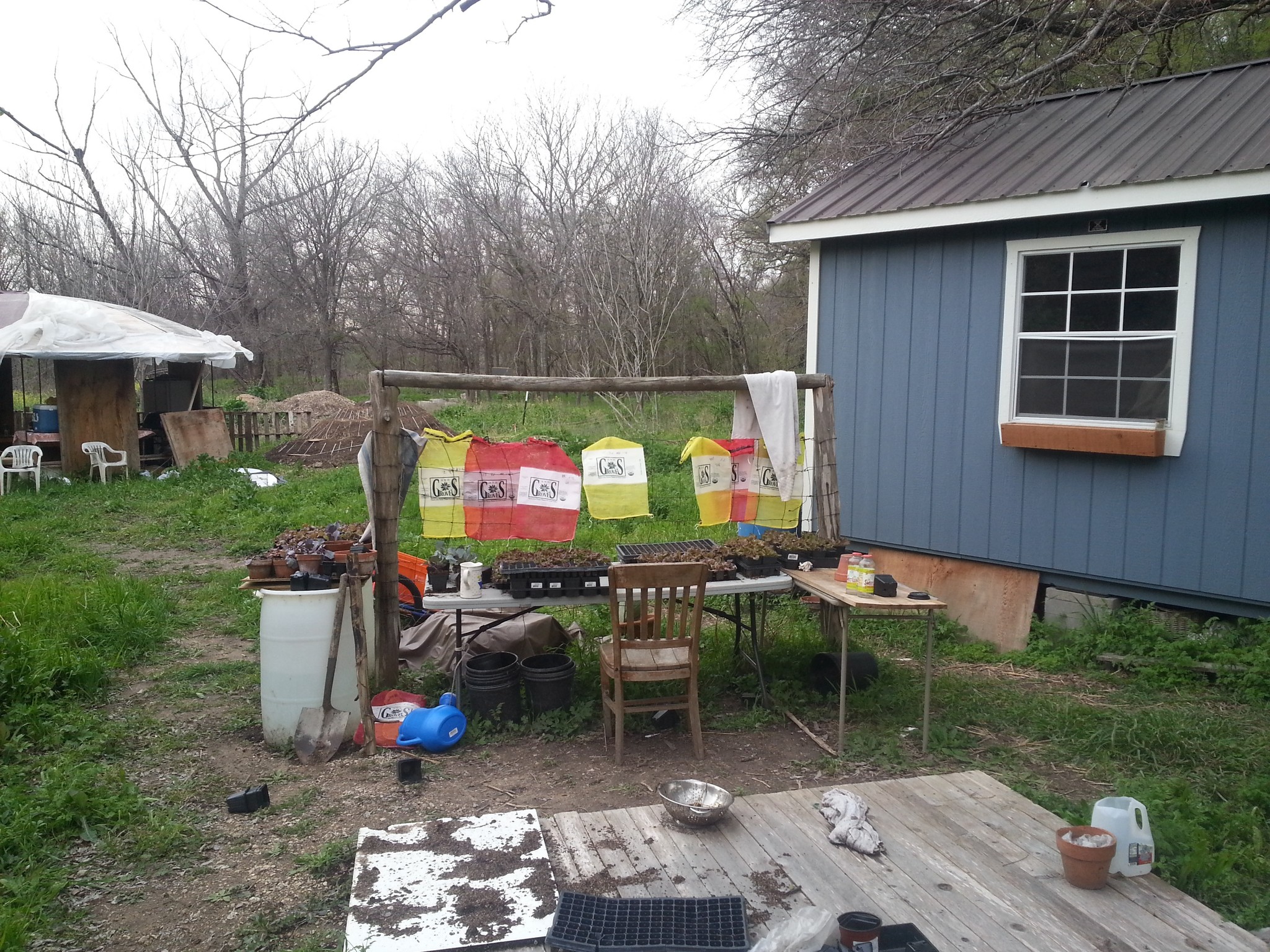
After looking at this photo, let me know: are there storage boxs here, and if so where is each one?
[32,403,59,433]
[370,547,428,604]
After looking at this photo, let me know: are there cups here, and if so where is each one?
[460,562,483,599]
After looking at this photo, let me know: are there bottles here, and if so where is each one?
[857,555,875,598]
[846,552,862,595]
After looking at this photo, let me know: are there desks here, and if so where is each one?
[758,563,949,758]
[12,429,155,469]
[420,567,795,714]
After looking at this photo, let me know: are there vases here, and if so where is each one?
[1056,823,1117,891]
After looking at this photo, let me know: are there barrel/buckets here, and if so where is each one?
[837,911,882,952]
[1056,826,1117,890]
[321,561,346,577]
[809,651,879,700]
[465,651,521,733]
[652,710,678,731]
[520,653,576,715]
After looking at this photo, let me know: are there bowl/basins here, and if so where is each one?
[334,550,378,576]
[834,554,852,582]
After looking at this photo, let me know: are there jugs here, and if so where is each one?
[396,692,467,752]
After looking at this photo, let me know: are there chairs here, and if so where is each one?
[0,445,45,496]
[81,440,130,485]
[596,561,710,762]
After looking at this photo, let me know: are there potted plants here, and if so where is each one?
[246,516,378,579]
[634,528,850,580]
